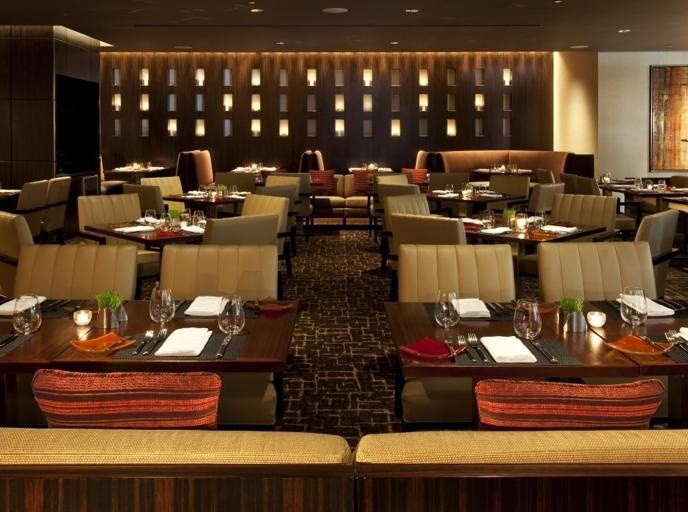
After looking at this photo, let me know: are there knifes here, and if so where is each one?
[104,335,133,351]
[216,334,232,358]
[530,340,557,363]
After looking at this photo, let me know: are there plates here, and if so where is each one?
[395,335,468,361]
[69,330,137,358]
[607,333,677,358]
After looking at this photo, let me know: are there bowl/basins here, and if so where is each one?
[72,310,94,325]
[586,311,608,327]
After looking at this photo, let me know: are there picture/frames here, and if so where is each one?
[648,64,688,174]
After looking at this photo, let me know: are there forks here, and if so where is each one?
[132,328,154,355]
[486,302,503,315]
[494,302,511,313]
[467,333,489,362]
[457,335,476,362]
[665,332,688,353]
[142,329,167,356]
[669,329,688,347]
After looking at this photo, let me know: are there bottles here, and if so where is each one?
[198,184,228,199]
[482,211,495,229]
[479,184,487,197]
[193,211,206,229]
[514,213,529,232]
[128,161,152,170]
[159,213,172,232]
[180,208,191,226]
[435,290,462,327]
[12,294,42,334]
[148,288,175,324]
[144,209,157,225]
[513,299,543,339]
[534,210,547,234]
[619,287,647,325]
[230,185,238,198]
[217,294,245,335]
[445,184,454,196]
[634,176,642,191]
[490,162,518,174]
[658,180,666,192]
